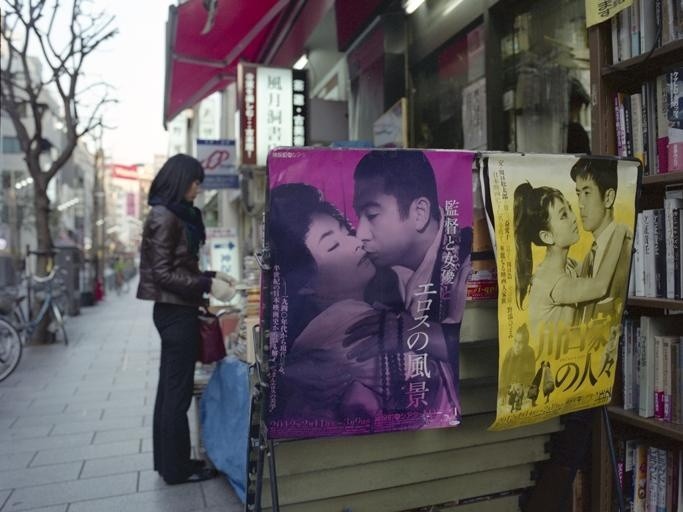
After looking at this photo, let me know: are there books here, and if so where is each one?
[609,0,683,511]
[193,254,261,399]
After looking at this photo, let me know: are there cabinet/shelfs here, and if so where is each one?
[246,144,641,512]
[593,1,682,511]
[403,16,491,151]
[486,1,594,156]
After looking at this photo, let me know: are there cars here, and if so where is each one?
[0,252,18,318]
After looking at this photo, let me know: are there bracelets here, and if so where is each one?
[428,322,434,359]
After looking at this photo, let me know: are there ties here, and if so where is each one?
[581,241,597,323]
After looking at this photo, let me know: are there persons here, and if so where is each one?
[508,385,518,413]
[568,157,633,329]
[611,331,617,356]
[133,153,238,486]
[515,389,522,411]
[542,361,555,404]
[512,178,627,328]
[500,323,536,400]
[566,76,593,154]
[605,338,616,362]
[526,361,546,407]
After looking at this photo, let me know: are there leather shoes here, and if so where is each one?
[159,459,219,485]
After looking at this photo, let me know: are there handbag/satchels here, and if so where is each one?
[196,310,226,364]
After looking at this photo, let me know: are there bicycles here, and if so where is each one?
[0,264,71,348]
[0,290,23,385]
[110,257,134,298]
